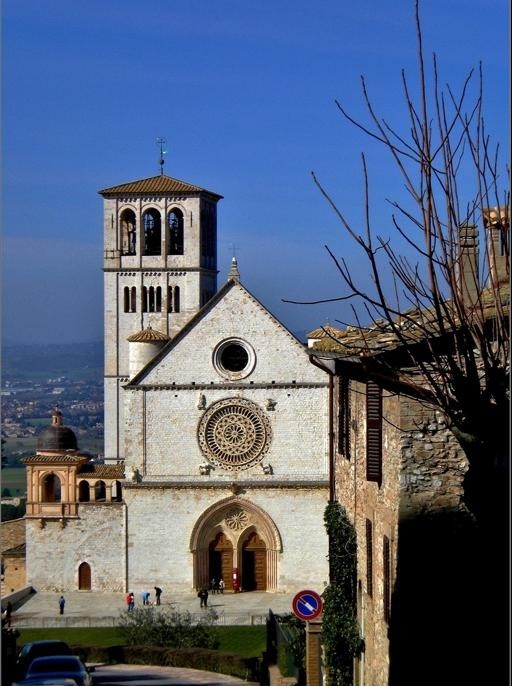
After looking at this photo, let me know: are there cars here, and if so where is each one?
[17,640,93,686]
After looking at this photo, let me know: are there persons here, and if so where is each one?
[5,601,13,628]
[210,578,218,595]
[141,592,151,606]
[219,578,225,593]
[59,595,66,615]
[131,593,135,611]
[127,593,133,613]
[203,589,209,607]
[154,587,162,606]
[197,589,204,608]
